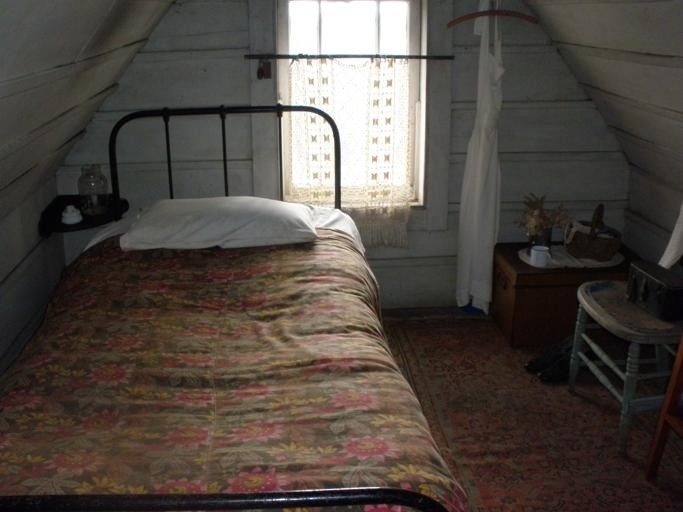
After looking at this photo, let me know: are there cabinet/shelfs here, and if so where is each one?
[493,242,642,344]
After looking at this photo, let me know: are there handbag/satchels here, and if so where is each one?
[562,218,621,262]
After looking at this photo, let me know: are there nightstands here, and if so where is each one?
[39,195,129,292]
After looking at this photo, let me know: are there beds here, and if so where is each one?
[0,105,475,512]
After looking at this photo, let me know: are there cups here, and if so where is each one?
[529,245,551,268]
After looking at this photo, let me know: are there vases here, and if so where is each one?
[527,227,552,255]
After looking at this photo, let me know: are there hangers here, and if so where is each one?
[447,0,538,28]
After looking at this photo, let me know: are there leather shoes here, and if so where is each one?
[526,342,602,385]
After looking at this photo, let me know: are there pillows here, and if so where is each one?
[120,196,316,251]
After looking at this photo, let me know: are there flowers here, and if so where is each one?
[511,192,572,236]
